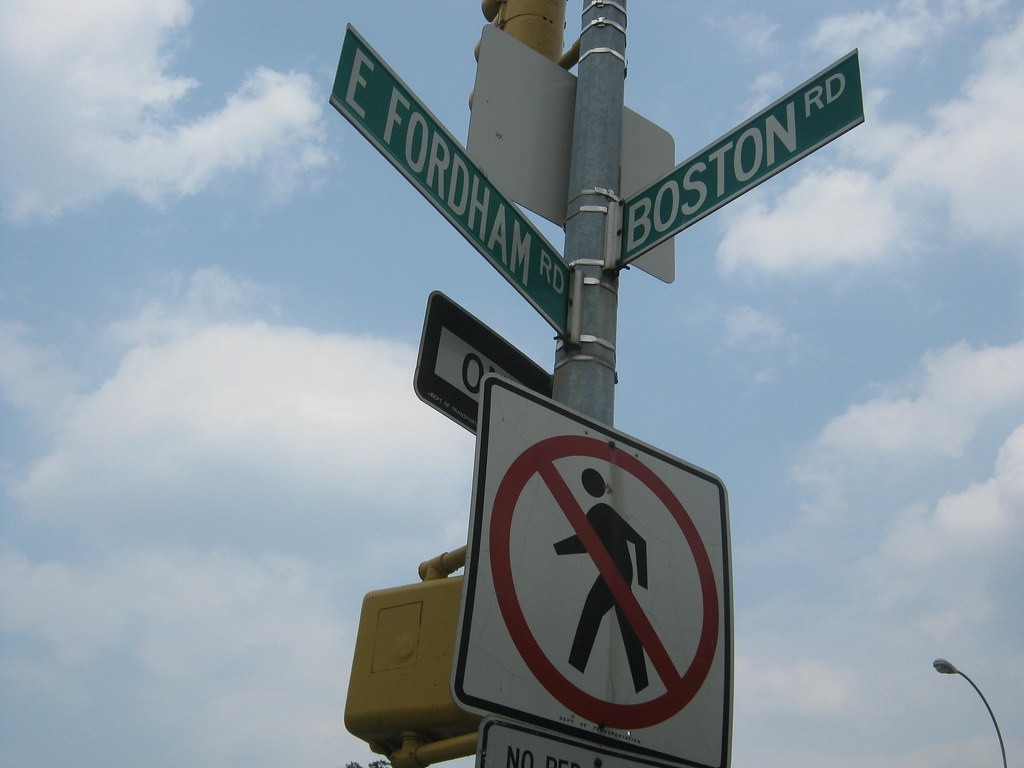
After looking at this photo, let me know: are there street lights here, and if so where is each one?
[933,658,1007,767]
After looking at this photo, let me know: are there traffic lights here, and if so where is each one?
[468,0,566,112]
